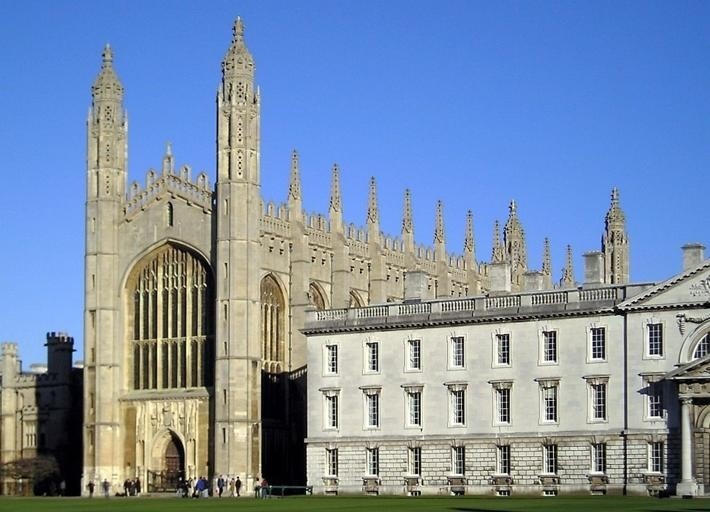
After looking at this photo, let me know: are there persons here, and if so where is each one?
[43,479,66,497]
[124,477,141,497]
[102,478,110,497]
[88,481,96,497]
[176,475,241,500]
[254,477,269,500]
